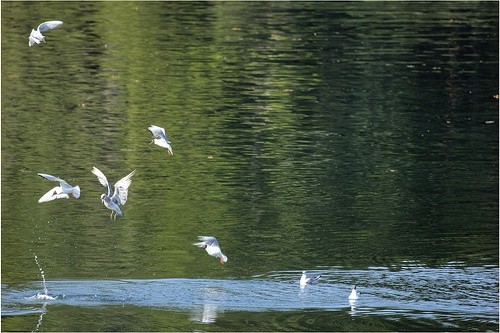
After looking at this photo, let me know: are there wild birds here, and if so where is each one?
[90,165,135,220]
[28,20,63,46]
[147,124,173,155]
[37,173,80,203]
[192,235,228,266]
[300,271,321,286]
[348,287,361,299]
